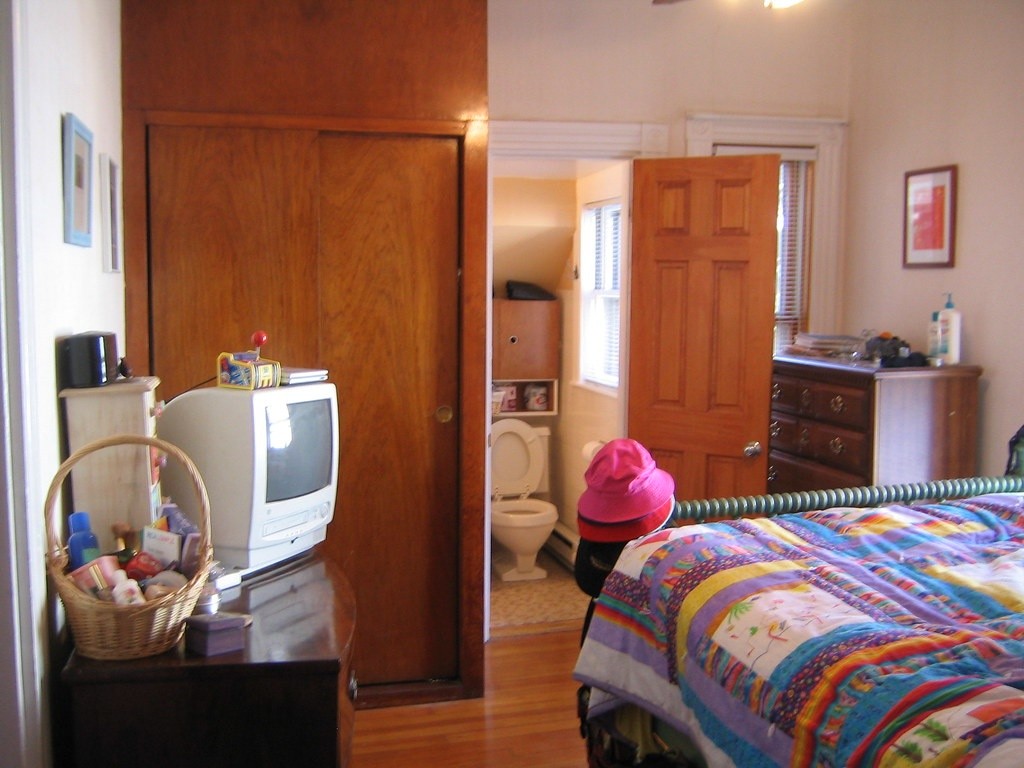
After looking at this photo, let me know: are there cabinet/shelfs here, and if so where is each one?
[767,354,982,496]
[51,554,361,768]
[490,297,563,417]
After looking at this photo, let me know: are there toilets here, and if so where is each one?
[490,419,559,581]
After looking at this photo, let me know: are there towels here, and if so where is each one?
[525,385,547,411]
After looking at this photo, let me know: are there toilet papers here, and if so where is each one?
[581,441,605,474]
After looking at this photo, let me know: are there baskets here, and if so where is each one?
[45,434,212,660]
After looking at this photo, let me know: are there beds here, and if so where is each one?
[572,465,1023,768]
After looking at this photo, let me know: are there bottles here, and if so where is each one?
[928,311,939,358]
[111,569,146,606]
[939,292,961,364]
[68,512,101,570]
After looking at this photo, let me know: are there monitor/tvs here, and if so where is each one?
[157,381,339,577]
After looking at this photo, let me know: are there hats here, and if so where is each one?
[579,438,675,543]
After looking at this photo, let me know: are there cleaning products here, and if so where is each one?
[927,292,960,364]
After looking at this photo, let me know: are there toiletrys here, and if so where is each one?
[64,502,204,602]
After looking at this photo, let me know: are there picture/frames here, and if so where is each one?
[900,163,957,270]
[100,155,125,277]
[62,113,95,247]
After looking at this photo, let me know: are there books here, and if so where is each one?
[784,334,867,357]
